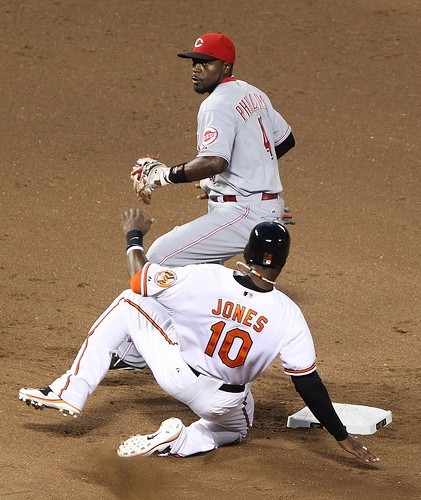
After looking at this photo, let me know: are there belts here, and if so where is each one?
[188,364,245,393]
[210,193,278,202]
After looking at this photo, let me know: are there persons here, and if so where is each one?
[19,209,381,464]
[108,33,295,371]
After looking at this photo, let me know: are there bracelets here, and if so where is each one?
[170,162,191,184]
[126,229,144,253]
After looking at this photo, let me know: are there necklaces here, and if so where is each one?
[236,261,276,287]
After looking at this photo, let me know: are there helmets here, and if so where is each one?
[245,222,290,268]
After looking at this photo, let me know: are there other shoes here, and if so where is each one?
[109,353,134,371]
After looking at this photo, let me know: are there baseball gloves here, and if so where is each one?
[130,157,171,203]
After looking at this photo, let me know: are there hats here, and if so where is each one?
[178,31,235,64]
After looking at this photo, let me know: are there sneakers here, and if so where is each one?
[19,386,81,418]
[117,417,183,457]
